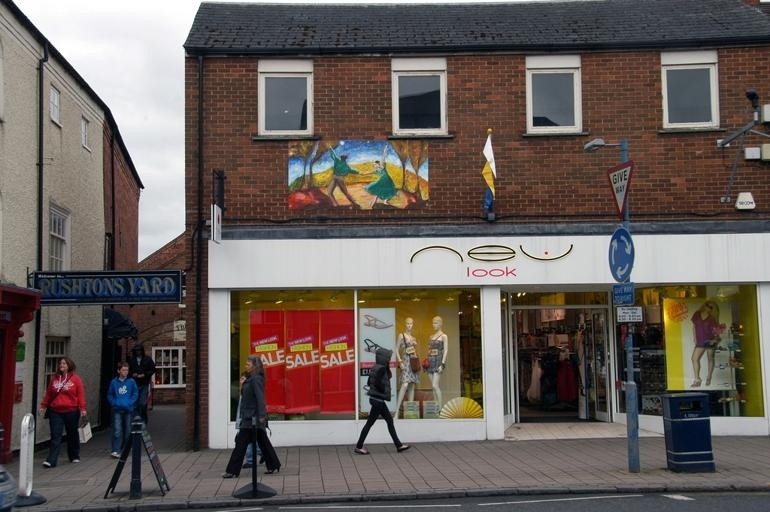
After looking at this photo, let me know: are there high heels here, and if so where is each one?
[223,472,239,478]
[691,377,702,387]
[265,464,280,474]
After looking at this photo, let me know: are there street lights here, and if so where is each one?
[581,136,643,475]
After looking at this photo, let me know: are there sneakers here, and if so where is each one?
[42,460,53,467]
[244,463,253,468]
[73,458,80,462]
[260,452,265,464]
[397,445,410,452]
[355,447,370,454]
[111,451,120,458]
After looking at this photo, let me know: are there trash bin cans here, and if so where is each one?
[661,392,716,473]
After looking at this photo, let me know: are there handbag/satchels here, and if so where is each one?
[43,407,50,419]
[411,357,420,372]
[78,415,92,444]
[252,415,268,428]
[422,359,430,369]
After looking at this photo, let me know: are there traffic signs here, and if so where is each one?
[616,303,644,323]
[611,282,635,307]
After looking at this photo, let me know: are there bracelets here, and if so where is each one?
[440,363,445,369]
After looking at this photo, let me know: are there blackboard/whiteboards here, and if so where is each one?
[142,430,168,486]
[109,428,132,488]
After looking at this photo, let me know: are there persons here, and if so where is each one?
[361,142,395,208]
[689,299,721,387]
[125,344,157,427]
[107,361,139,458]
[354,348,412,454]
[234,371,267,468]
[220,355,282,478]
[326,142,364,209]
[393,318,420,419]
[38,358,89,468]
[423,315,449,415]
